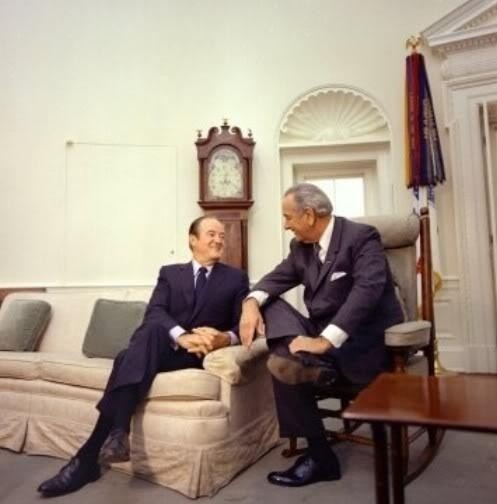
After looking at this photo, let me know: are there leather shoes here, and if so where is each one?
[266,353,338,389]
[102,429,129,462]
[266,452,340,487]
[37,455,101,495]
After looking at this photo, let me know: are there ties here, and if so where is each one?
[196,267,207,289]
[309,244,323,289]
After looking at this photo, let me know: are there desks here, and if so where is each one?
[341,373,496,504]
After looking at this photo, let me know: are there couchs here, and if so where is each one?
[0,293,288,499]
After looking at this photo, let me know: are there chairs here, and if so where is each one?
[282,206,448,486]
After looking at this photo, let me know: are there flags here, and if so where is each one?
[400,37,446,208]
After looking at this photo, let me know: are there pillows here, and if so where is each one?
[82,298,147,359]
[0,298,51,352]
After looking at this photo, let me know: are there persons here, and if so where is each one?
[239,183,406,487]
[38,215,253,495]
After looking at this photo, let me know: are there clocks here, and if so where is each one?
[194,117,256,273]
